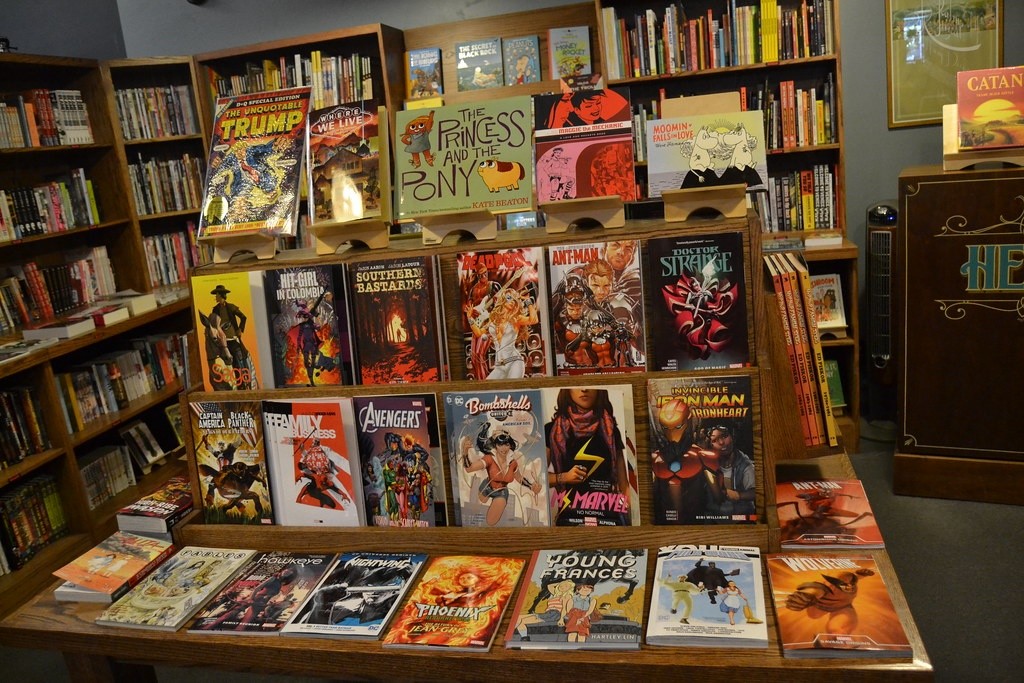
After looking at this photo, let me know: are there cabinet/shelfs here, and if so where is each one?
[0,0,937,683]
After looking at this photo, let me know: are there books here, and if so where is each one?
[0,0,916,664]
[955,65,1024,150]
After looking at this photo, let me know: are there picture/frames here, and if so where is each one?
[885,0,1003,129]
